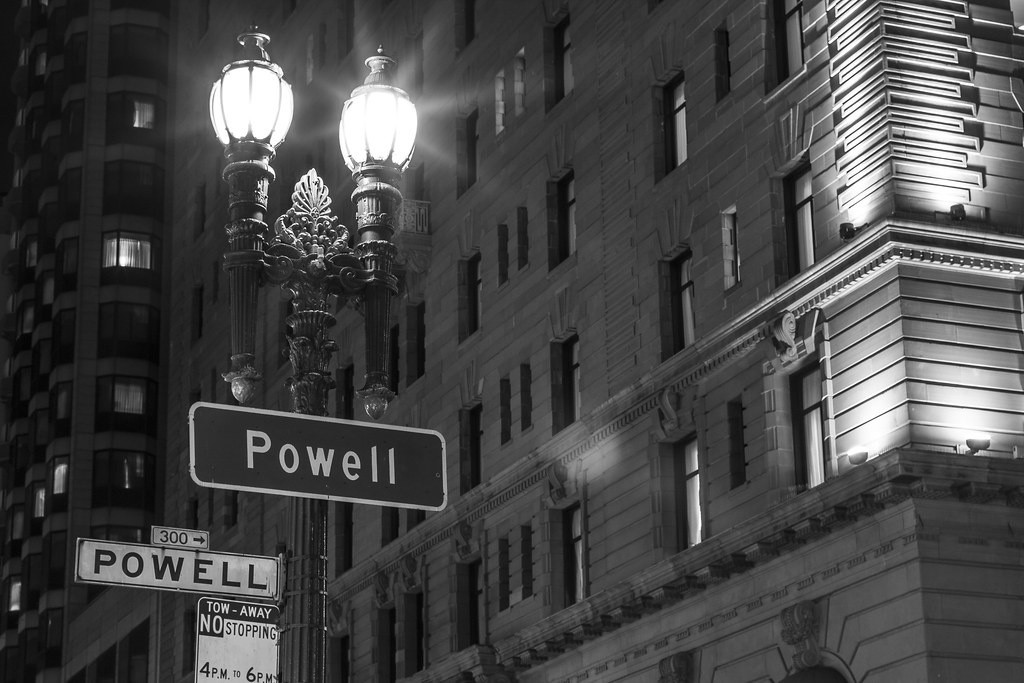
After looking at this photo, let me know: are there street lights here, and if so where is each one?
[209,18,421,682]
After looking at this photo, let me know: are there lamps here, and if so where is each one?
[849,452,868,466]
[956,437,990,457]
[950,204,966,222]
[839,220,867,241]
